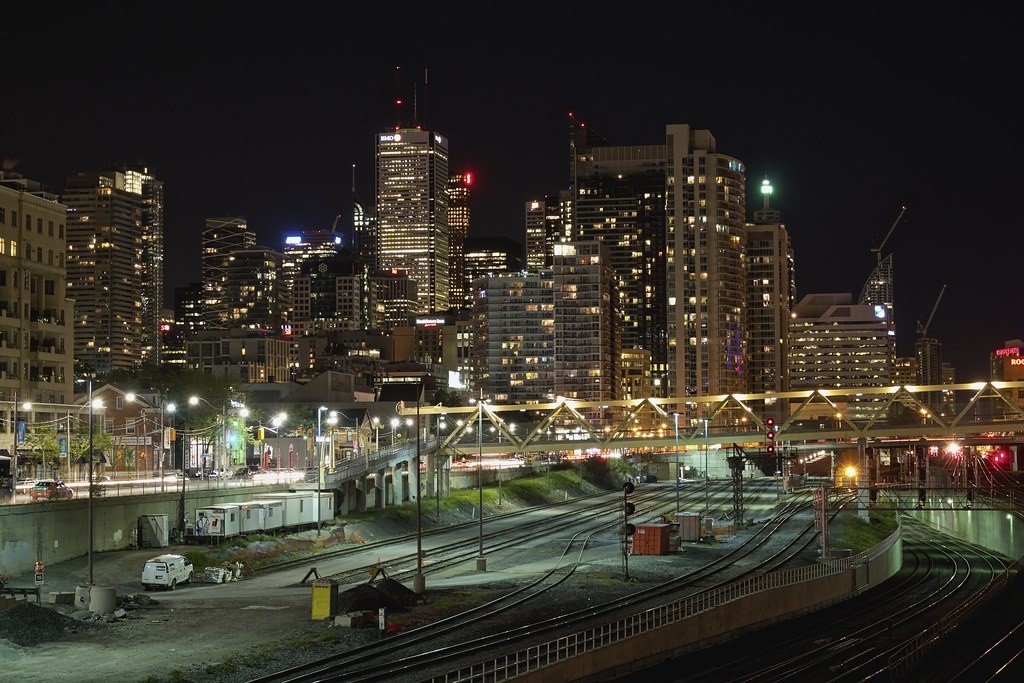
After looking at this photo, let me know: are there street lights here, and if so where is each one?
[317,406,328,466]
[259,413,288,470]
[668,411,685,522]
[10,390,32,502]
[330,411,380,458]
[188,396,250,488]
[65,399,103,483]
[391,418,413,447]
[469,388,493,560]
[125,392,175,494]
[76,367,101,587]
[701,418,712,518]
[272,418,281,476]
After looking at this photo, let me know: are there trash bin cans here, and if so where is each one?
[311,578,341,620]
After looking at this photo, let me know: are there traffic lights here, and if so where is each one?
[767,431,775,440]
[767,446,774,453]
[766,417,774,427]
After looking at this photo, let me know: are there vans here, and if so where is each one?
[140,553,194,591]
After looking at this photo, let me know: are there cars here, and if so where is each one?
[29,480,73,500]
[234,468,251,479]
[250,466,261,474]
